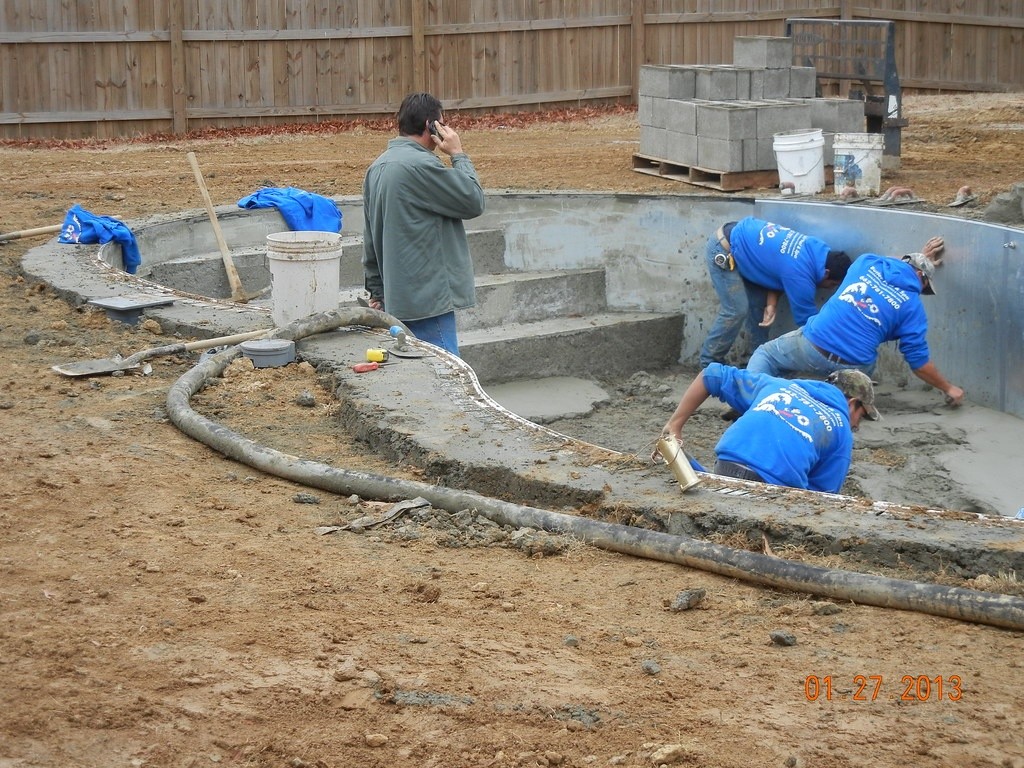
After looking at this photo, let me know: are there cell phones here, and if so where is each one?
[429,120,443,141]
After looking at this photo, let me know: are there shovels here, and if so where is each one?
[49,327,271,378]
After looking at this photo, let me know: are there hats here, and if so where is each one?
[825,368,879,421]
[901,253,936,295]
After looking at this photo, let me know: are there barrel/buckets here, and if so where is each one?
[266,230,342,330]
[832,132,885,197]
[772,128,826,194]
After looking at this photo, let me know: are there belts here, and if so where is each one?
[717,221,739,250]
[803,335,849,364]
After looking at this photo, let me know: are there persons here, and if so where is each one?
[746,236,965,408]
[661,362,885,494]
[699,216,852,369]
[361,92,486,358]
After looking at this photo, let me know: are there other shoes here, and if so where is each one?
[720,405,739,421]
